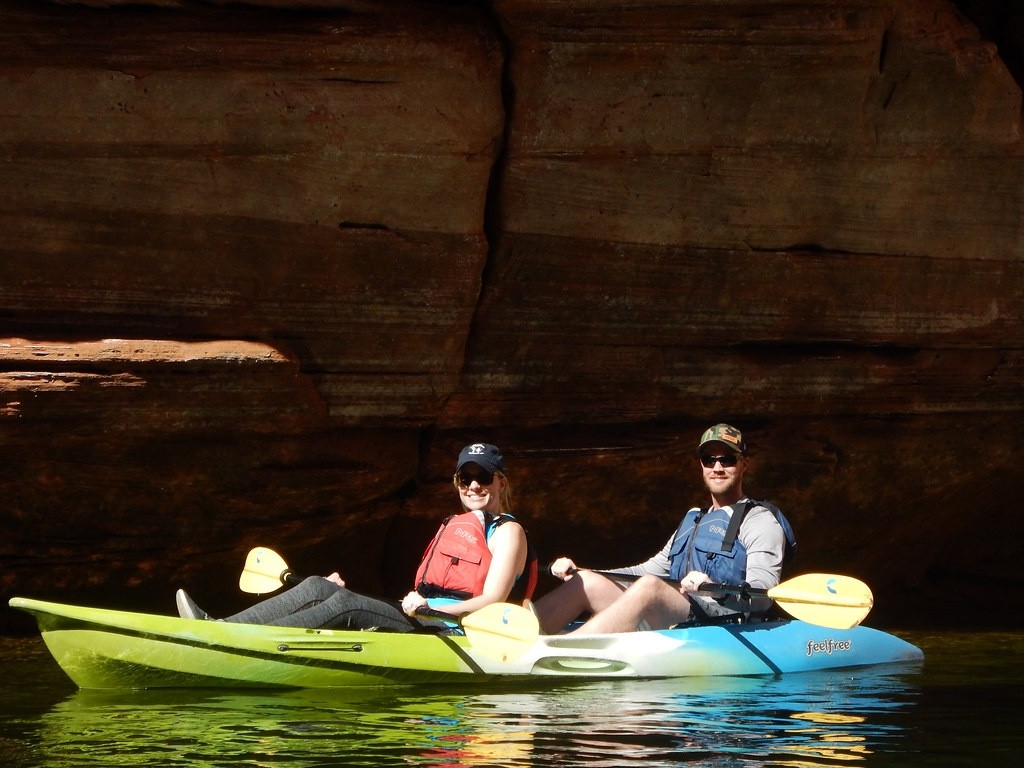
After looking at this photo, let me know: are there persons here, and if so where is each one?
[176,443,529,629]
[531,425,789,635]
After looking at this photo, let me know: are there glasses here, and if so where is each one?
[458,470,493,486]
[701,454,738,468]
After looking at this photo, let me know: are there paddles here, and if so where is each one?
[239,545,541,657]
[545,560,875,631]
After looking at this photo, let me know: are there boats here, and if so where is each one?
[8,596,926,694]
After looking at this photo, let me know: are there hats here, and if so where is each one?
[697,423,746,453]
[457,443,504,473]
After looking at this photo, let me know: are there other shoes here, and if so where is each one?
[522,599,547,635]
[176,588,215,622]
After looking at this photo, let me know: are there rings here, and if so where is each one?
[690,580,694,584]
